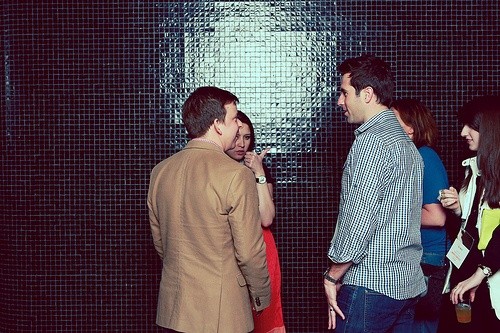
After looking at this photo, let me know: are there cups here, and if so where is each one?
[455,298,472,323]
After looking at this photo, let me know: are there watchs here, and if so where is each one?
[256,175,268,184]
[322,268,338,284]
[477,265,491,278]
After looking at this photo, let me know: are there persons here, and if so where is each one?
[223,109,287,333]
[389,98,448,333]
[146,86,271,333]
[440,97,500,333]
[322,56,427,333]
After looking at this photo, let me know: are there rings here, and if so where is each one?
[329,308,335,311]
[454,287,457,289]
